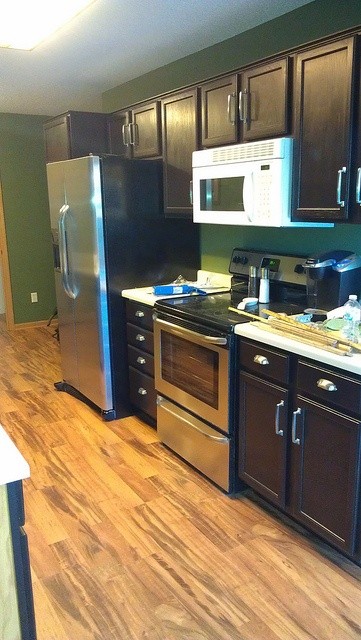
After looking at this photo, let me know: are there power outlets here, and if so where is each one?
[31,292,38,303]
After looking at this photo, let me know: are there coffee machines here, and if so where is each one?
[302,250,360,311]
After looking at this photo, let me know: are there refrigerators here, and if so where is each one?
[45,152,202,422]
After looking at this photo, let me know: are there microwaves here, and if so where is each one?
[191,137,334,229]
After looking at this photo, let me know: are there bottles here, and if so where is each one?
[343,295,360,344]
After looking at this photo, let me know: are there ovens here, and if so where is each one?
[152,311,249,495]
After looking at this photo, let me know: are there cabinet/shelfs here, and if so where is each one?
[161,87,199,214]
[201,56,289,146]
[292,33,361,223]
[237,340,361,557]
[120,289,155,421]
[106,101,161,159]
[42,111,109,163]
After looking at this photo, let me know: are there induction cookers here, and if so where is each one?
[153,247,317,334]
